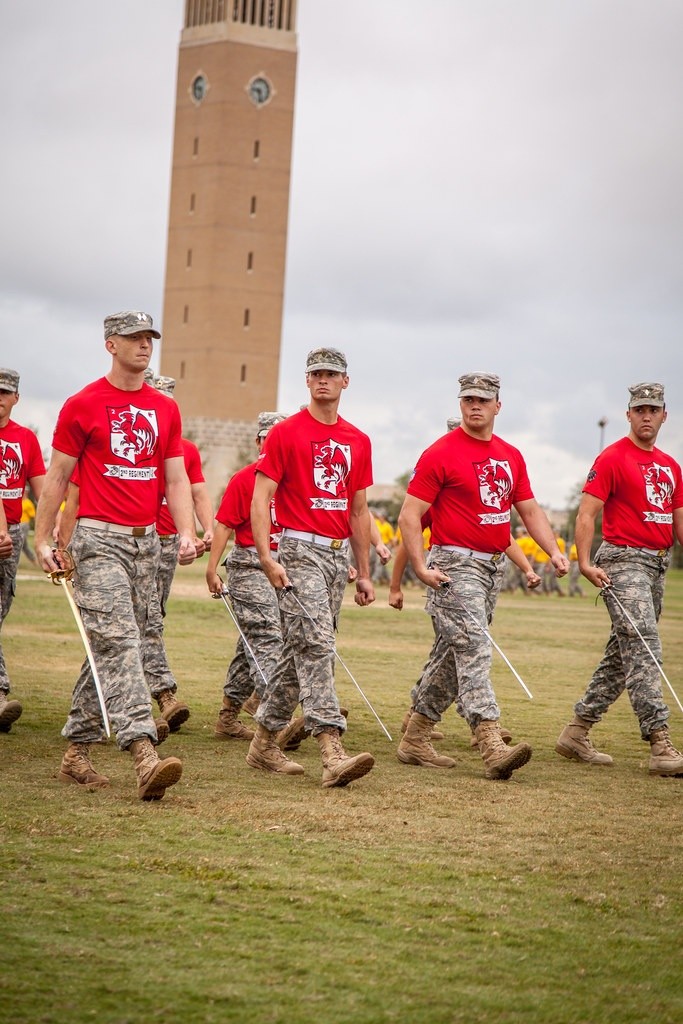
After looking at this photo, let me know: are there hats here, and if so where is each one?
[305,347,346,374]
[154,377,174,398]
[104,311,160,339]
[447,417,462,432]
[627,383,665,408]
[0,369,19,392]
[457,371,499,399]
[256,412,290,436]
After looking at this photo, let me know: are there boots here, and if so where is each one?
[152,717,168,747]
[275,715,310,751]
[316,727,374,788]
[400,706,443,740]
[214,696,255,740]
[471,721,511,750]
[246,723,304,774]
[648,728,683,776]
[553,714,614,764]
[59,741,110,788]
[474,721,532,779]
[241,693,260,715]
[155,690,189,731]
[396,713,456,768]
[129,738,182,801]
[0,692,21,733]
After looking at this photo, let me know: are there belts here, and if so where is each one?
[7,524,19,530]
[440,545,503,562]
[75,518,156,537]
[635,547,668,556]
[281,528,349,548]
[242,546,278,559]
[157,534,179,539]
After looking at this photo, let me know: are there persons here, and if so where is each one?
[145,368,213,746]
[508,527,586,597]
[205,410,354,746]
[36,310,196,802]
[390,418,541,747]
[399,373,569,779]
[369,506,431,591]
[555,383,683,776]
[243,512,390,718]
[250,346,375,782]
[0,369,61,733]
